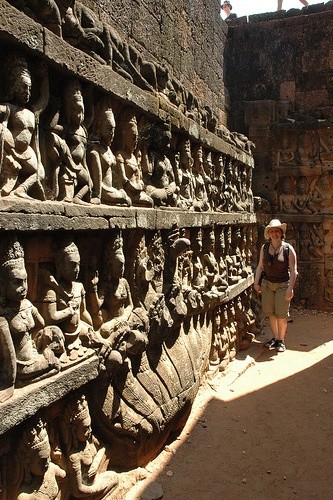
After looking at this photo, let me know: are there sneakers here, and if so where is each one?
[275,341,286,351]
[264,338,276,350]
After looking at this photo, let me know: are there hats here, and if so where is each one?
[264,218,287,239]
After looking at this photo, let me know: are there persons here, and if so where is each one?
[301,217,333,266]
[220,0,238,21]
[270,131,333,214]
[253,219,299,353]
[0,65,262,500]
[323,277,333,306]
